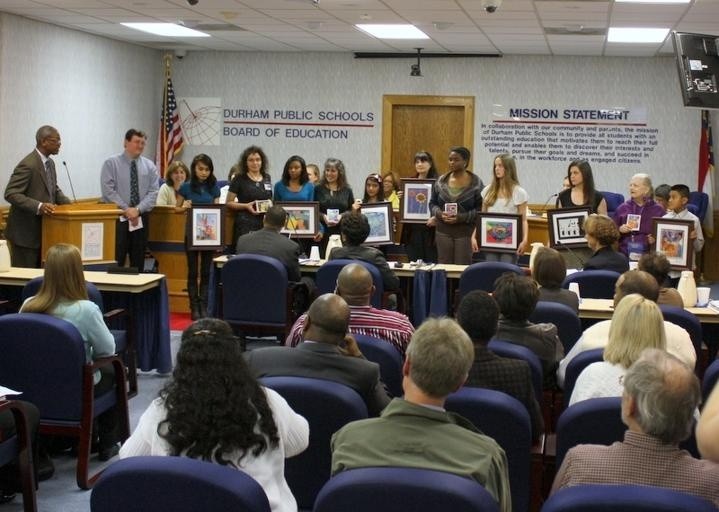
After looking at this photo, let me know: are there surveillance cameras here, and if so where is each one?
[480,0,502,14]
[411,64,420,75]
[175,49,186,59]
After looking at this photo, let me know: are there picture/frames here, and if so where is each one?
[547,206,593,250]
[399,177,437,224]
[188,201,224,252]
[651,217,695,272]
[355,202,394,247]
[272,201,320,240]
[475,213,524,253]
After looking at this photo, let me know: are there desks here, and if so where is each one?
[1,263,169,296]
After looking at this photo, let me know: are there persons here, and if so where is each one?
[117,262,565,512]
[101,129,159,272]
[397,146,529,265]
[547,254,719,507]
[156,152,221,320]
[534,246,579,313]
[555,158,704,280]
[19,242,121,463]
[3,126,70,268]
[219,145,399,311]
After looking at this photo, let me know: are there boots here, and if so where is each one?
[189,286,208,320]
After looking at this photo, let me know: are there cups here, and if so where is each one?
[309,245,321,265]
[629,260,640,270]
[568,282,580,304]
[698,286,711,306]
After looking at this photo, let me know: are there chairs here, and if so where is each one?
[242,257,719,468]
[597,192,624,218]
[1,398,42,512]
[89,452,271,510]
[213,250,292,341]
[539,483,719,510]
[22,274,138,401]
[1,311,133,490]
[218,179,230,191]
[157,177,165,186]
[312,466,504,512]
[687,191,709,273]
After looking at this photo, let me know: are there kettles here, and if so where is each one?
[676,269,698,309]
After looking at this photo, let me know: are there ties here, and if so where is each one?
[130,160,140,207]
[46,160,54,201]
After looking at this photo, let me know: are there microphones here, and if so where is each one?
[538,192,558,217]
[62,160,80,203]
[285,211,308,260]
[556,240,585,267]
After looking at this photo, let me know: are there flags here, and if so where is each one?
[154,74,185,180]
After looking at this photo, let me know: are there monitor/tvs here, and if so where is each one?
[668,29,718,108]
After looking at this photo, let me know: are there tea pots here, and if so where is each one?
[324,235,344,261]
